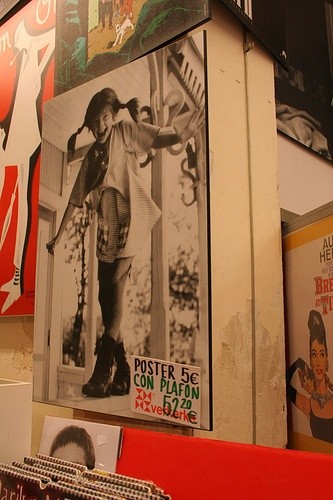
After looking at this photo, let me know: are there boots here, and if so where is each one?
[82,335,117,397]
[107,340,130,394]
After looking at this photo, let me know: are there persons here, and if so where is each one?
[120,0,134,21]
[45,87,206,396]
[286,310,333,443]
[49,425,97,470]
[97,0,114,32]
